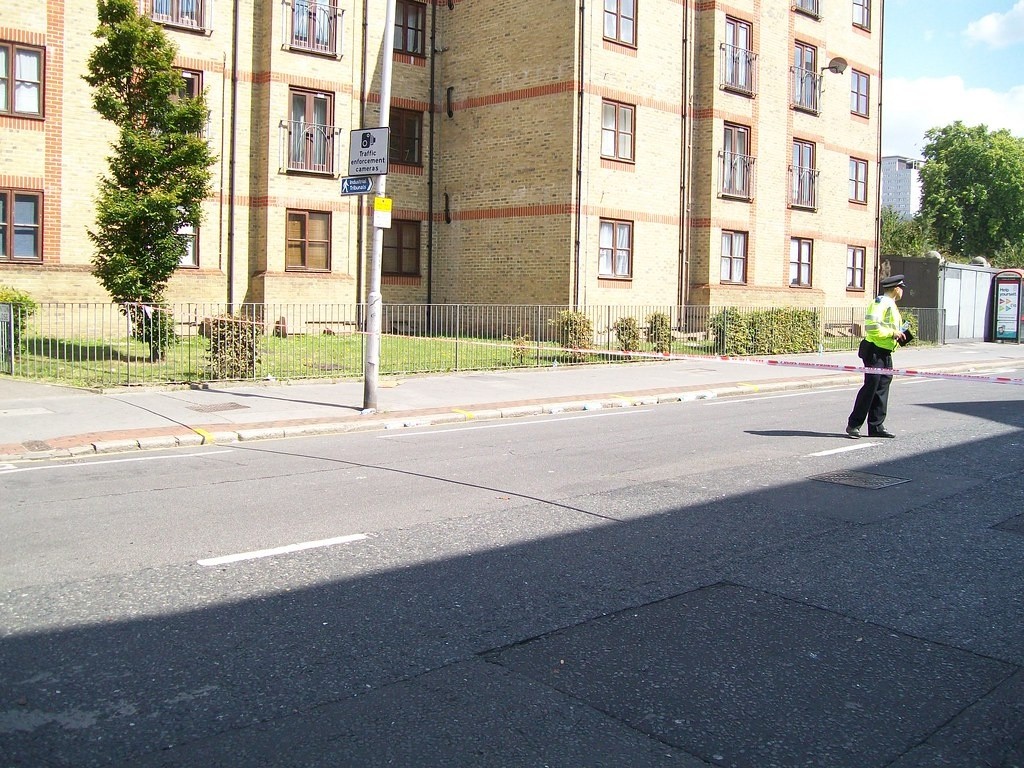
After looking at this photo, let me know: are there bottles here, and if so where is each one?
[897,321,910,340]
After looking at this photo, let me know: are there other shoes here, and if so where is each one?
[846,425,861,438]
[868,429,896,438]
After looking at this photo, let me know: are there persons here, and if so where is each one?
[846,274,906,438]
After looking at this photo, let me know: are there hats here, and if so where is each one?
[881,274,906,289]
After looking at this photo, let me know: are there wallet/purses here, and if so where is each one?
[898,330,913,346]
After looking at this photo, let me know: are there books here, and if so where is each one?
[897,329,914,347]
